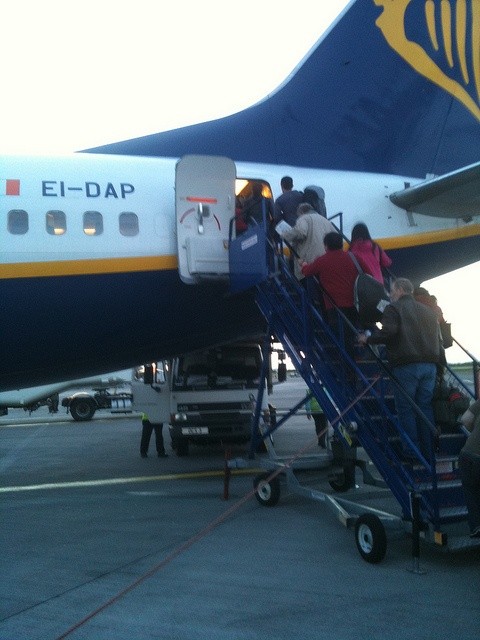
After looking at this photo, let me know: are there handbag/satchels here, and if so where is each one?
[445,385,469,418]
[441,322,452,348]
[353,273,391,322]
[432,374,453,425]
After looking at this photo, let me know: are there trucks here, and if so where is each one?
[130,337,287,460]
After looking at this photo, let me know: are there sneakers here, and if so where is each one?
[387,441,420,458]
[469,527,480,537]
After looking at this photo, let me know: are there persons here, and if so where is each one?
[268,176,305,276]
[355,277,444,465]
[346,223,392,360]
[47,214,55,235]
[296,231,357,364]
[236,196,247,237]
[242,182,282,276]
[413,287,447,400]
[140,414,169,458]
[304,373,333,449]
[457,397,480,537]
[280,202,339,320]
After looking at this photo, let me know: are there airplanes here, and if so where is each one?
[0,1,480,395]
